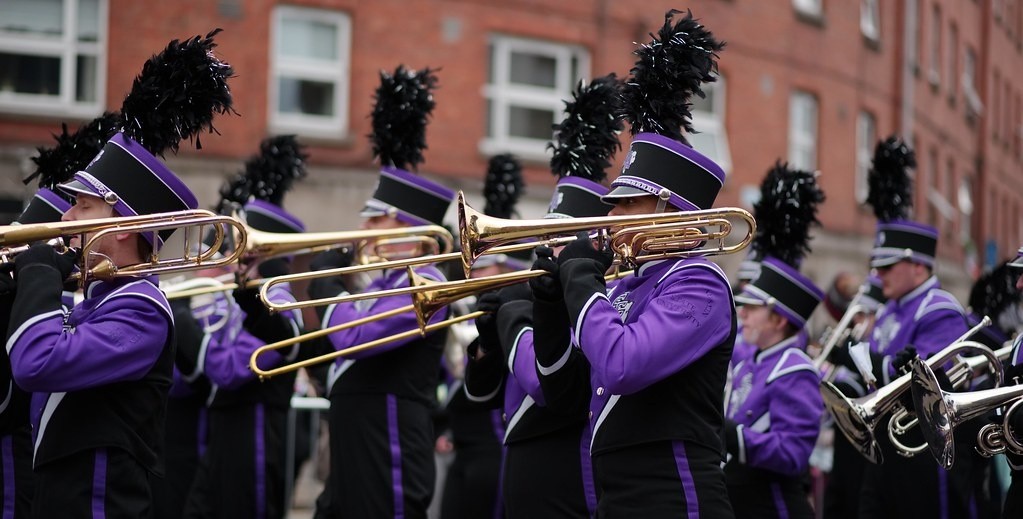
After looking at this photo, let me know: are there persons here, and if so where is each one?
[533,132,739,519]
[0,136,1023,519]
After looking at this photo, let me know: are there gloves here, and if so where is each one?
[529,246,568,321]
[307,250,350,322]
[558,233,614,269]
[233,283,294,358]
[173,306,205,351]
[720,417,742,457]
[475,290,499,355]
[499,283,534,305]
[9,239,81,281]
[892,346,919,410]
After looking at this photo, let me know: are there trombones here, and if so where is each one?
[230,189,759,377]
[814,283,874,382]
[817,314,1023,472]
[0,208,248,288]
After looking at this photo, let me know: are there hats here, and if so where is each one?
[224,134,311,265]
[540,73,624,217]
[854,269,888,312]
[10,110,123,225]
[197,155,267,262]
[469,152,537,270]
[864,133,937,269]
[57,27,241,247]
[355,63,456,226]
[733,170,825,329]
[600,9,726,210]
[737,159,801,279]
